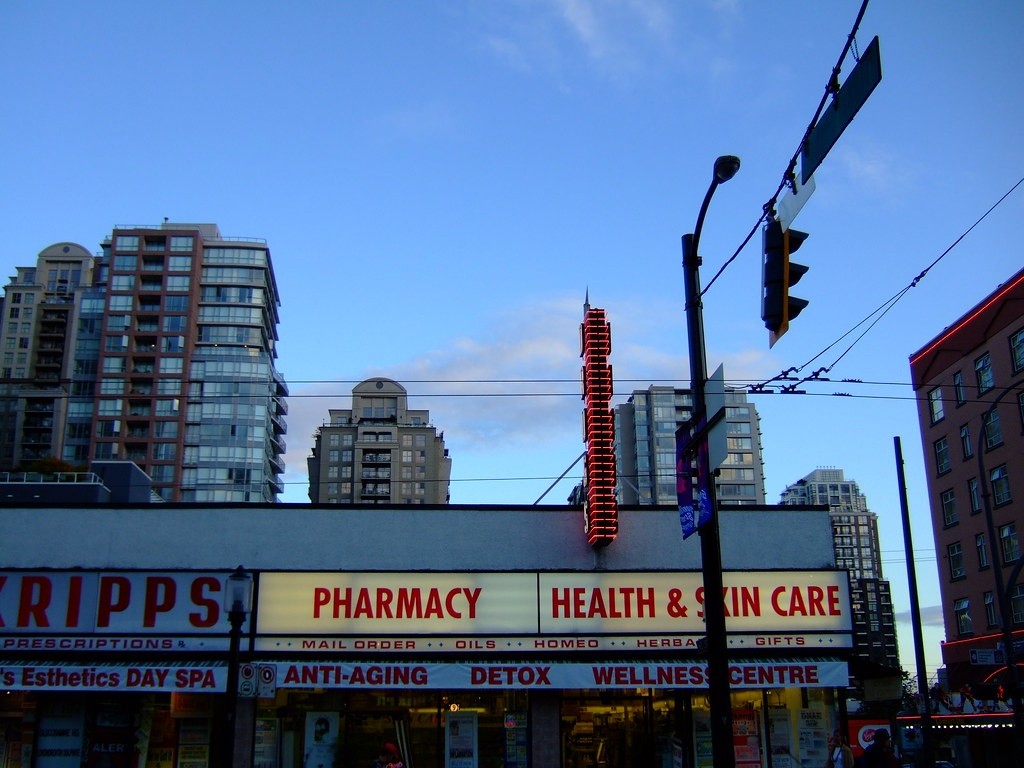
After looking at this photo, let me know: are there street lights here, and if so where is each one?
[220,565,255,768]
[679,155,741,768]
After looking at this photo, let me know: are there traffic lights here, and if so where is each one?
[762,216,809,349]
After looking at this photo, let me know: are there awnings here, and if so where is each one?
[0,646,240,698]
[244,650,851,697]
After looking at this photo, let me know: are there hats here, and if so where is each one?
[872,728,892,739]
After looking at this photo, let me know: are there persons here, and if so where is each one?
[374,743,403,768]
[824,728,854,768]
[853,728,900,768]
